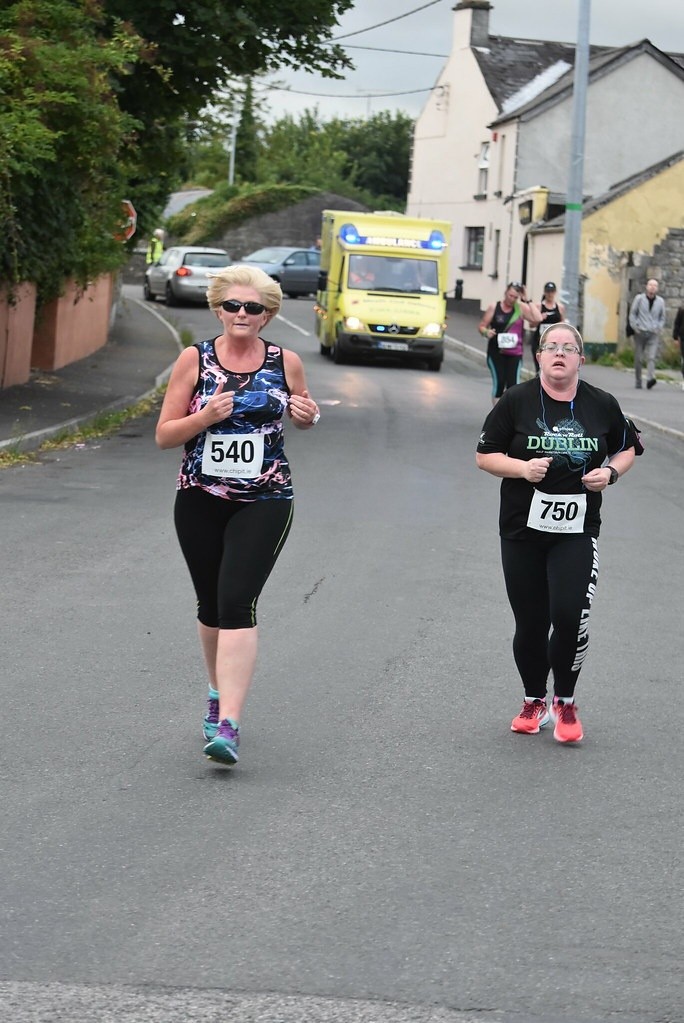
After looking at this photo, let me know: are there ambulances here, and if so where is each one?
[314,209,451,370]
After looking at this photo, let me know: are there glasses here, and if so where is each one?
[540,344,582,356]
[218,299,268,315]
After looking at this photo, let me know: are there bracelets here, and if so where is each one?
[527,299,532,303]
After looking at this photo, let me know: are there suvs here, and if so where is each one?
[144,246,232,305]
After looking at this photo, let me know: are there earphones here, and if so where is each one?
[578,363,581,368]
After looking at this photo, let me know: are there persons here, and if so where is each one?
[530,281,565,378]
[146,228,164,271]
[476,323,645,742]
[155,264,320,765]
[478,281,543,409]
[673,301,684,393]
[629,278,666,389]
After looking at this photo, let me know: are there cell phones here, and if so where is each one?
[625,417,631,429]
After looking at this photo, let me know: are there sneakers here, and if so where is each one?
[511,697,549,734]
[549,694,583,744]
[203,681,219,741]
[203,717,240,765]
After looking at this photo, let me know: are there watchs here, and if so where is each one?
[310,410,320,426]
[603,466,619,485]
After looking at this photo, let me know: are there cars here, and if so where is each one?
[236,245,322,298]
